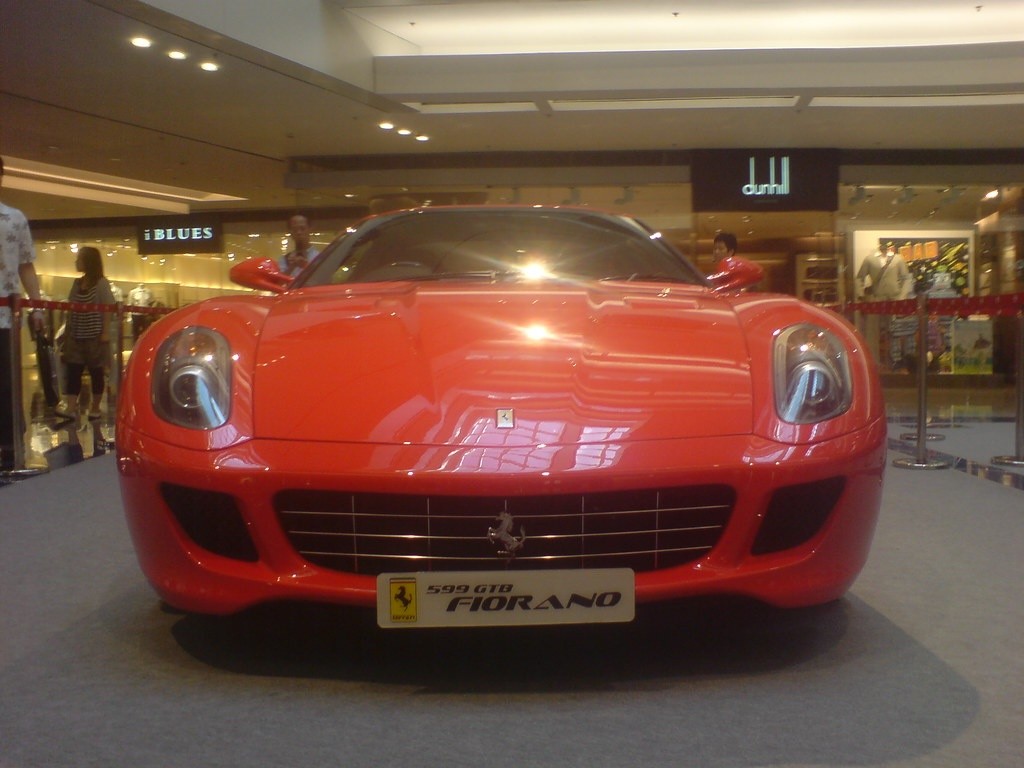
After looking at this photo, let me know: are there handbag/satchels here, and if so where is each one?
[61,340,86,369]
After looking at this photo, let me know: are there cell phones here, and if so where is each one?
[296,251,303,256]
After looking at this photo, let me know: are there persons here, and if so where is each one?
[110,282,154,345]
[277,212,332,290]
[60,246,115,420]
[0,157,45,486]
[53,420,105,464]
[857,244,942,370]
[714,235,738,265]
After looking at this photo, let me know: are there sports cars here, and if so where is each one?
[111,202,889,633]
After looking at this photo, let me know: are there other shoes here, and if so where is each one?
[88,410,102,420]
[56,408,77,420]
